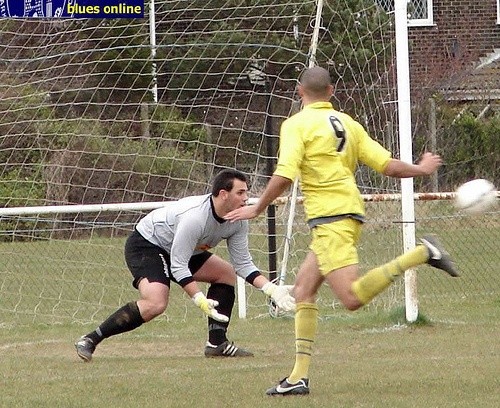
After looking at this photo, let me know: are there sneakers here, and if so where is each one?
[74,334,96,363]
[205,340,254,358]
[416,234,460,277]
[266,376,310,396]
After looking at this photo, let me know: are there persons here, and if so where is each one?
[222,66,462,396]
[75,168,296,363]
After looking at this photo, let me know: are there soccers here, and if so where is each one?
[455,178,497,217]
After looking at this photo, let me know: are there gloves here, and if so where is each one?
[190,291,229,323]
[261,282,296,312]
